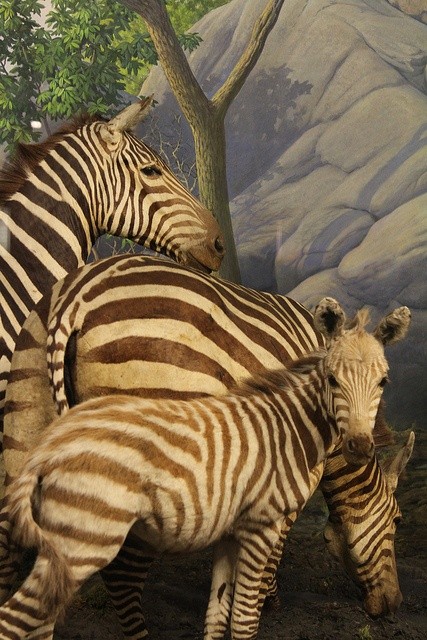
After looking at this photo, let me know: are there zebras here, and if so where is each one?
[0,93,226,639]
